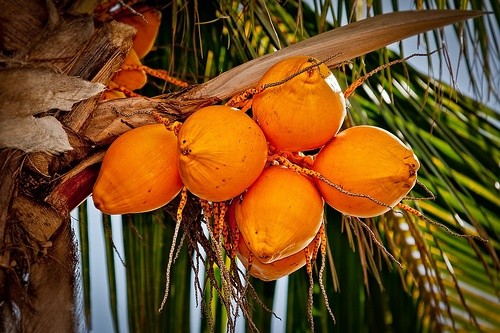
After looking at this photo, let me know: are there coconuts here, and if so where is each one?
[90,1,421,282]
